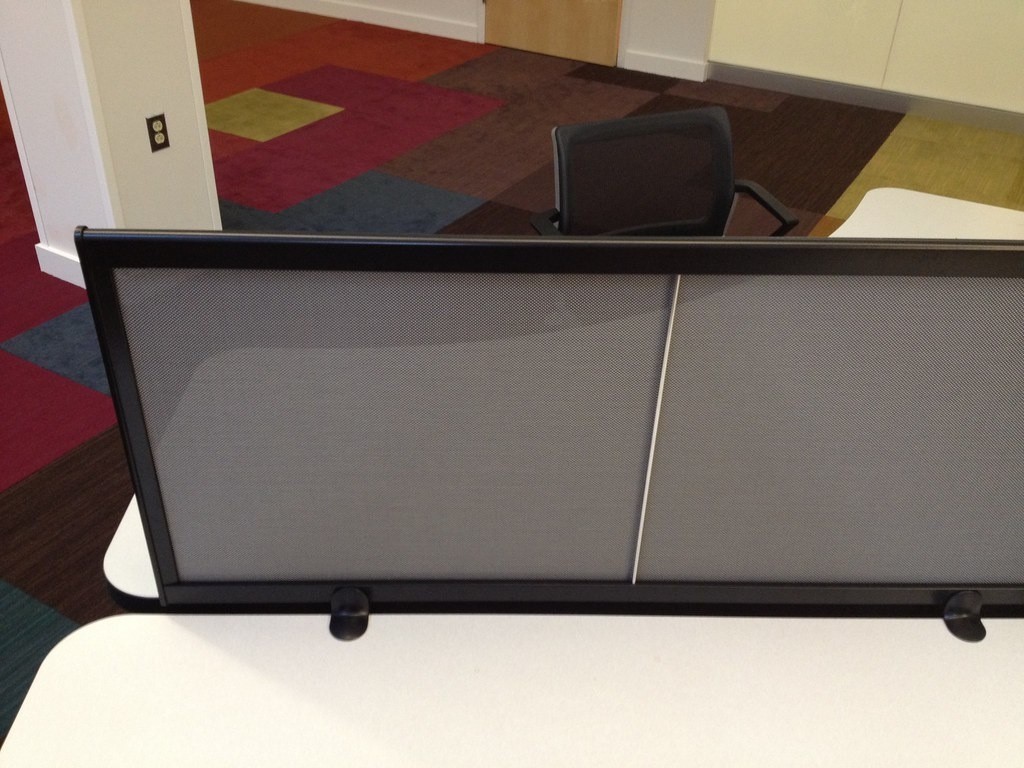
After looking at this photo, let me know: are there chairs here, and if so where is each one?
[530,106,800,236]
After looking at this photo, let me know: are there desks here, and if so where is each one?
[0,188,1024,768]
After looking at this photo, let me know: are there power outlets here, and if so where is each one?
[146,113,170,153]
